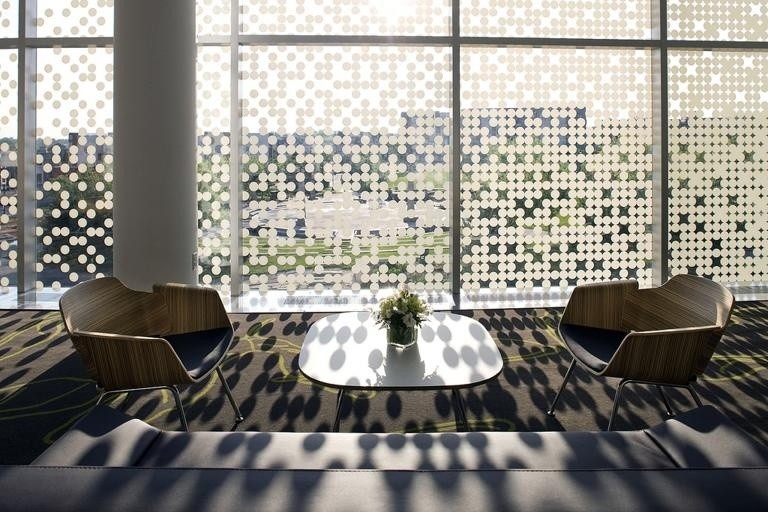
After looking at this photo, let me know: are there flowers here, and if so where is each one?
[372,288,433,344]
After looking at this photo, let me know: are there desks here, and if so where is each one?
[297,311,503,432]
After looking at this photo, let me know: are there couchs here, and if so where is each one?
[1,404,768,512]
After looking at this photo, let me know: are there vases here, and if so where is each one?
[386,326,418,348]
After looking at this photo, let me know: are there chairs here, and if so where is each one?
[547,274,734,431]
[60,276,243,432]
